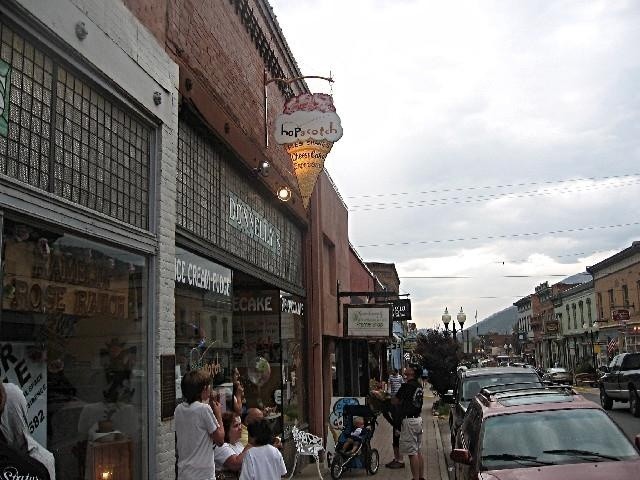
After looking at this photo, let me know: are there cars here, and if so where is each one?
[448,366,544,451]
[541,368,573,386]
[450,381,640,480]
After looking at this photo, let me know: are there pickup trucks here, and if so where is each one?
[597,352,640,417]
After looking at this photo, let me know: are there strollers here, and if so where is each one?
[331,403,379,479]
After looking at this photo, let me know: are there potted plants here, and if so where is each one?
[99,408,117,433]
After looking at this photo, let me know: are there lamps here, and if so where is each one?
[249,159,271,176]
[276,186,295,203]
[593,319,608,327]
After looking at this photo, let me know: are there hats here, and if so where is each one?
[408,364,423,376]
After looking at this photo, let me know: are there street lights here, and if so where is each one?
[583,321,600,388]
[441,305,466,367]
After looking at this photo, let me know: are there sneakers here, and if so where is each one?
[385,459,405,469]
[339,449,351,456]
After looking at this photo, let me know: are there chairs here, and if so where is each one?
[289,425,331,480]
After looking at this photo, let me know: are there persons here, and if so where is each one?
[173,366,288,480]
[369,362,428,480]
[47,367,142,480]
[340,417,372,455]
[0,382,57,480]
